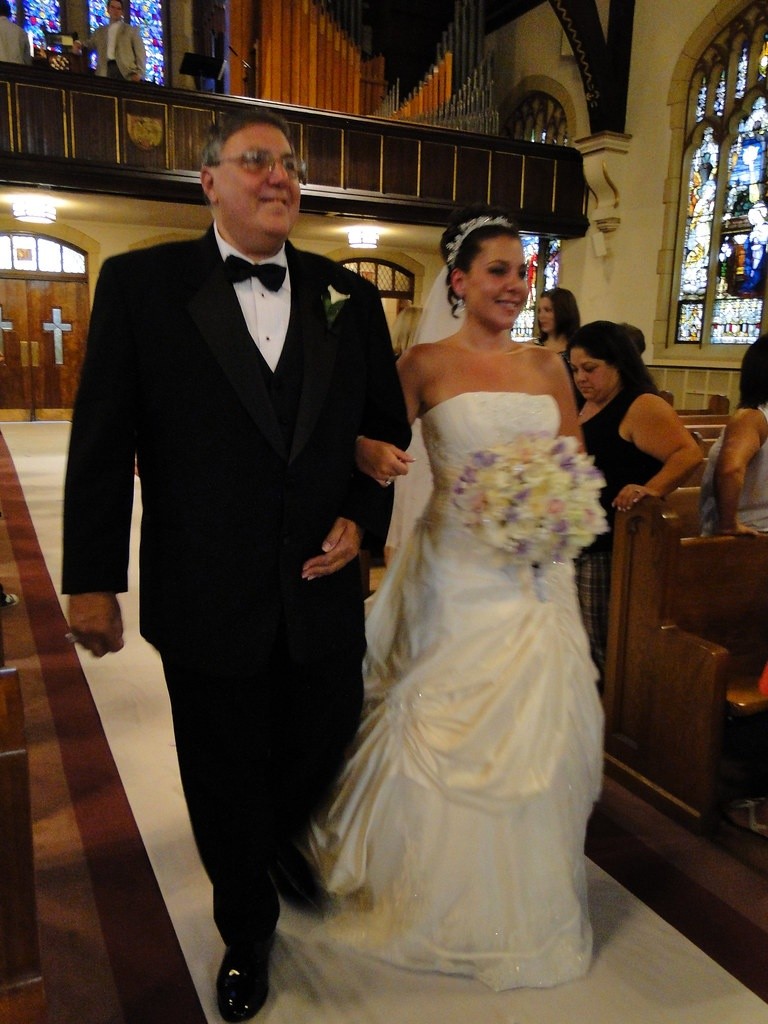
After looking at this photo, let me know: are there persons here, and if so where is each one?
[71,0,147,82]
[343,205,604,994]
[0,0,36,67]
[59,109,413,1024]
[698,332,768,537]
[525,286,705,698]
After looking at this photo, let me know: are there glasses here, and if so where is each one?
[209,145,303,177]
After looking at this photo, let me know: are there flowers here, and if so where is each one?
[323,285,351,324]
[449,433,610,600]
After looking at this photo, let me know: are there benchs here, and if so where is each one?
[602,388,768,876]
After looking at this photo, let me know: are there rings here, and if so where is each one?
[634,488,640,493]
[63,632,79,644]
[384,476,393,485]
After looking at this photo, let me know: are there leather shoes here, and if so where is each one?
[215,937,271,1023]
[271,846,332,914]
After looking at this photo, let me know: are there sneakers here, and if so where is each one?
[4,594,21,608]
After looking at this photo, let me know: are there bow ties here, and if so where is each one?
[223,254,287,293]
